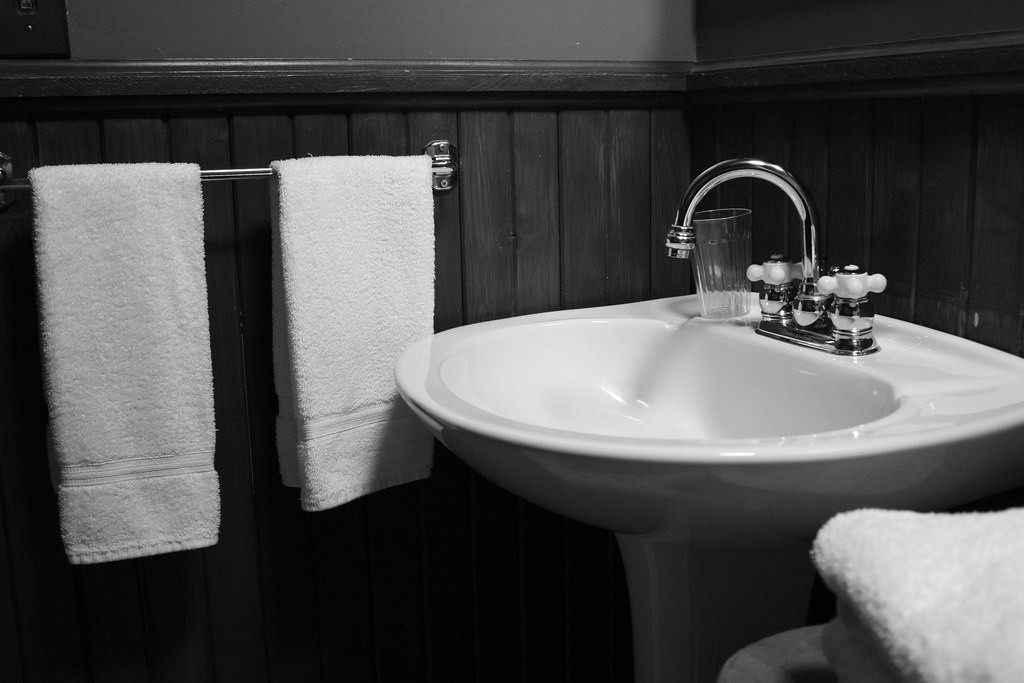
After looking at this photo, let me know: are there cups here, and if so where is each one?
[690,207,752,318]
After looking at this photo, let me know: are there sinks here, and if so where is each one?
[394,292,1024,548]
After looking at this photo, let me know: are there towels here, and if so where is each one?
[267,152,441,514]
[25,160,224,567]
[807,505,1024,683]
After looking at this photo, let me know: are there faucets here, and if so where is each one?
[664,155,889,358]
